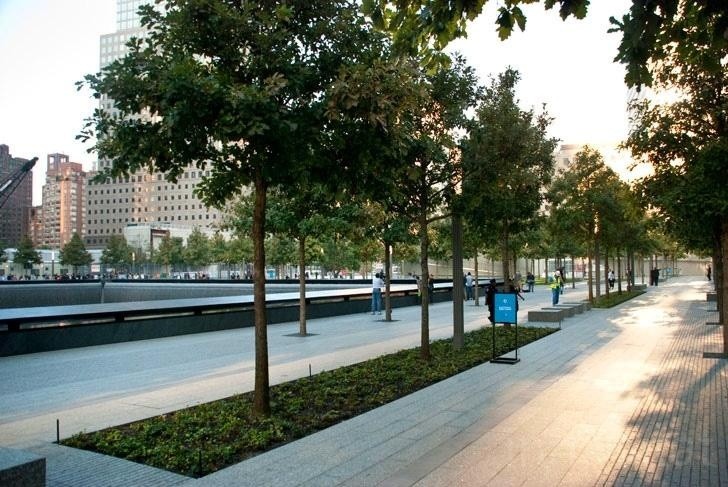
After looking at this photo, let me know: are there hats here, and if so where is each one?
[489,279,496,282]
[554,271,561,277]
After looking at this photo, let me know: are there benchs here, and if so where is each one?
[527,301,593,321]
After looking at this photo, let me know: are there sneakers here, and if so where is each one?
[370,312,382,315]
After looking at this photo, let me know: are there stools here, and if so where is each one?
[0,447,46,487]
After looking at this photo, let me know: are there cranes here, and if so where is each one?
[0,157,38,208]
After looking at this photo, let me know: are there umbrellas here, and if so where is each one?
[557,266,565,270]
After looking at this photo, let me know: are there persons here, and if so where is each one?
[415,276,422,305]
[611,270,616,289]
[607,268,614,289]
[371,272,385,314]
[653,267,659,285]
[1,266,256,281]
[428,273,435,303]
[485,279,501,322]
[706,264,711,280]
[465,271,475,299]
[509,270,567,310]
